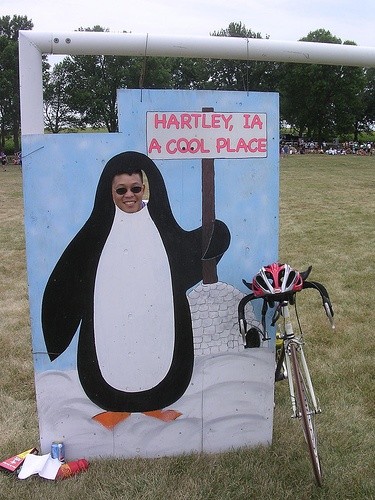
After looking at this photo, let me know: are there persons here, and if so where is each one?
[0,150,21,172]
[279,132,374,158]
[113,167,149,213]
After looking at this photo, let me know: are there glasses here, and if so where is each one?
[113,187,143,194]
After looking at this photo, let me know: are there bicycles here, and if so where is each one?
[238,266,333,489]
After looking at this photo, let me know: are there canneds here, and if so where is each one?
[51,441,64,464]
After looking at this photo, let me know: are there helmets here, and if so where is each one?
[251,262,302,297]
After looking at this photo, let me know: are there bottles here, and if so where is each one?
[57,458,90,480]
[276,324,284,364]
